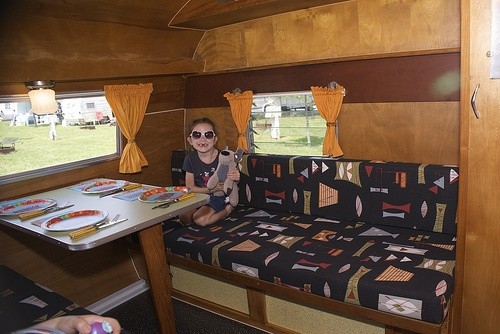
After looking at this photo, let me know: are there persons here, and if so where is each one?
[11,315,122,334]
[181,117,240,227]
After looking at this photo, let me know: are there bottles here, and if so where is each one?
[90,320,113,334]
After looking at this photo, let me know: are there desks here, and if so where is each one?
[0,177,211,334]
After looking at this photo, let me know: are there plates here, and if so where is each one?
[138,185,192,203]
[0,198,58,216]
[41,208,109,233]
[81,179,129,194]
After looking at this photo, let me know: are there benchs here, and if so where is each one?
[163,150,459,333]
[0,265,125,334]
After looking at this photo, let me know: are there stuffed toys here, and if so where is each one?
[207,145,244,207]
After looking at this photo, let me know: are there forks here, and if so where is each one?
[68,214,121,236]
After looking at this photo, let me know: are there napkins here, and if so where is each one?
[67,178,96,192]
[0,197,29,209]
[31,211,71,227]
[112,186,154,201]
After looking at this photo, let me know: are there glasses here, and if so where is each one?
[189,130,215,140]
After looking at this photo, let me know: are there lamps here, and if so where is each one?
[25,81,59,114]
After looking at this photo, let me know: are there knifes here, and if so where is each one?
[152,194,195,209]
[70,218,128,239]
[20,204,74,220]
